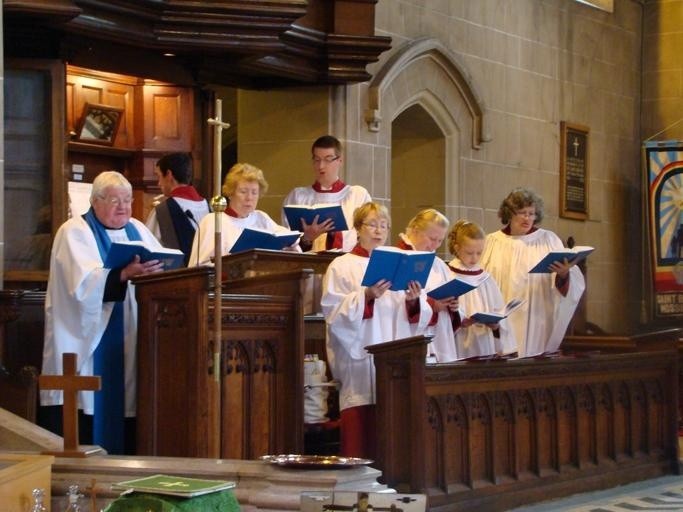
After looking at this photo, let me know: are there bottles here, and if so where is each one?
[31,488,47,512]
[65,482,85,512]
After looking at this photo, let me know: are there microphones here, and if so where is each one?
[185,209,201,267]
[566,236,575,248]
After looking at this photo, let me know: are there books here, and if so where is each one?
[526,245,598,272]
[103,241,184,270]
[227,229,300,253]
[361,246,436,291]
[283,203,350,233]
[469,298,526,324]
[425,277,491,300]
[109,473,236,498]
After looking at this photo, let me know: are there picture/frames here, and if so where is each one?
[75,101,125,147]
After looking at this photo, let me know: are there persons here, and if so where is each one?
[478,189,585,358]
[281,134,371,254]
[145,153,211,266]
[40,170,164,455]
[394,209,463,364]
[186,164,303,266]
[445,218,518,358]
[319,201,433,458]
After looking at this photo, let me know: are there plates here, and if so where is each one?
[258,452,374,470]
